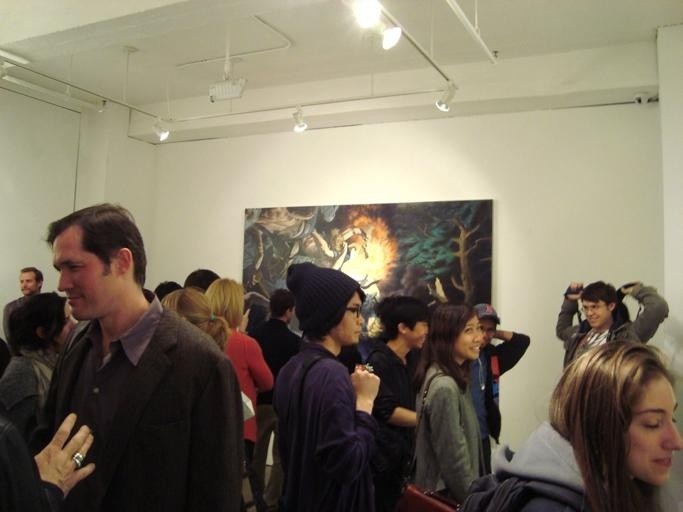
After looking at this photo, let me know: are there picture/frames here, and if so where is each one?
[240,199,494,306]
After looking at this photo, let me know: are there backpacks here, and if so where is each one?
[563,281,644,365]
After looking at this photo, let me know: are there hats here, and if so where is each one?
[284,261,360,333]
[474,304,499,325]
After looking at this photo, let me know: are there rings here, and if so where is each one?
[72,452,85,471]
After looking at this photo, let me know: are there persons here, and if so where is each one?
[20,267,44,295]
[205,279,273,471]
[245,288,302,510]
[470,304,530,476]
[366,296,429,512]
[460,340,683,511]
[272,263,380,511]
[556,282,669,371]
[2,291,76,433]
[1,413,96,512]
[41,204,244,512]
[160,290,228,354]
[409,301,484,503]
[182,270,251,334]
[153,282,184,301]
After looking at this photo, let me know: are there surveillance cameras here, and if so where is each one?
[634,92,648,105]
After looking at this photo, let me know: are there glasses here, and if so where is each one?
[580,303,609,311]
[343,304,361,317]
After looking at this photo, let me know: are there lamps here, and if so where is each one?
[371,19,403,50]
[152,117,170,141]
[434,79,457,112]
[293,104,307,132]
